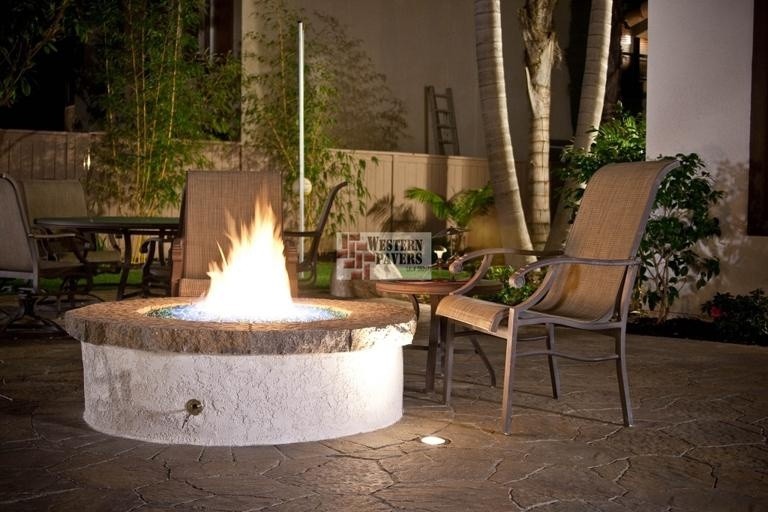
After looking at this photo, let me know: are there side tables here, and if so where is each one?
[377,277,505,396]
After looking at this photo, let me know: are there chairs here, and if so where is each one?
[279,180,349,291]
[434,154,682,435]
[0,171,186,315]
